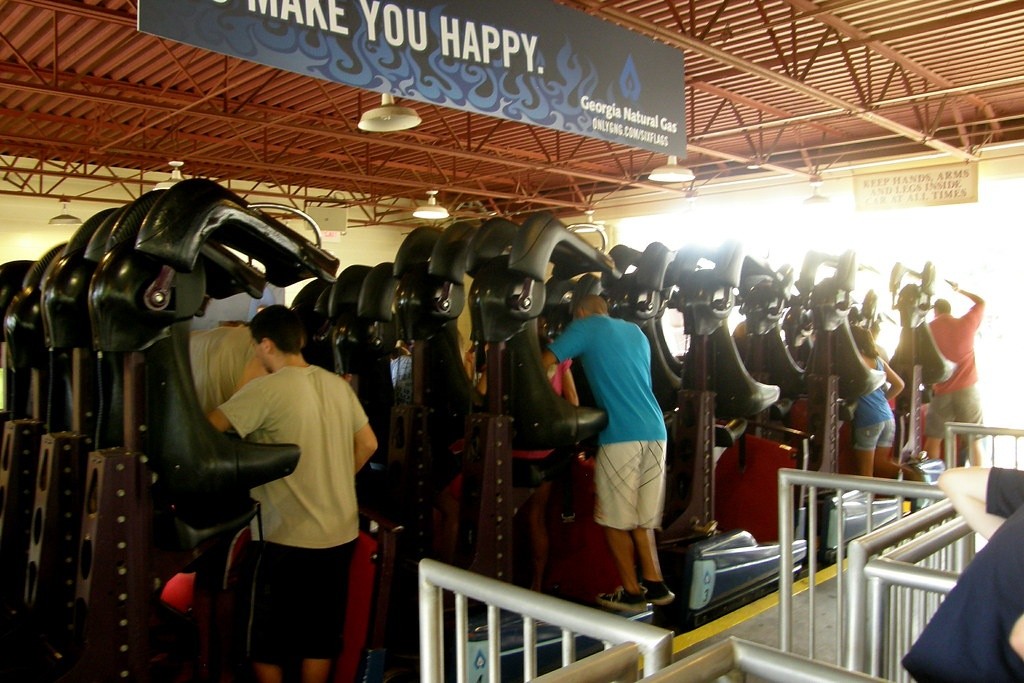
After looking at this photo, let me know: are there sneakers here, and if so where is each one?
[590,585,647,612]
[638,585,676,603]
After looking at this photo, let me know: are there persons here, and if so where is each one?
[922,279,988,465]
[547,291,677,613]
[512,315,580,593]
[850,322,913,477]
[207,303,377,682]
[902,467,1023,683]
